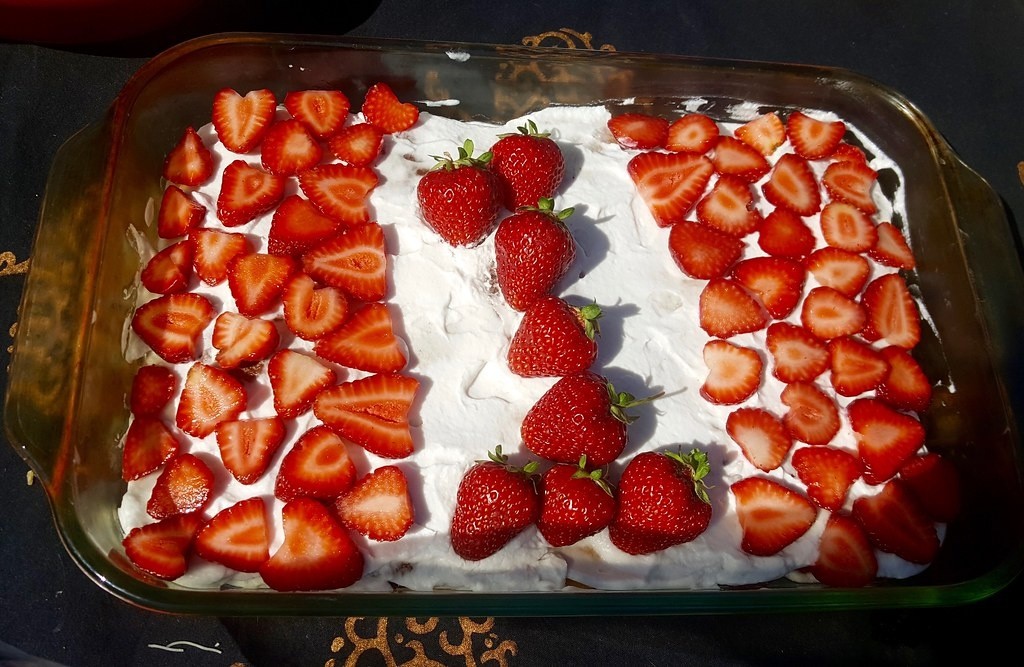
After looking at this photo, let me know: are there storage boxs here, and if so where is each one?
[2,28,1021,618]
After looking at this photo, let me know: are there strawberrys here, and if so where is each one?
[121,83,961,591]
